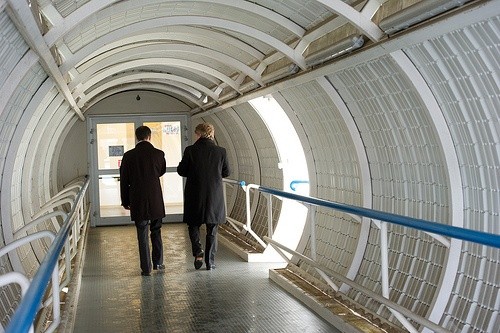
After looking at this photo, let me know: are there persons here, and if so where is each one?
[119,126,167,276]
[177,123,231,271]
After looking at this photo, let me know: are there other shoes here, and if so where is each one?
[141,271,151,276]
[194,249,204,269]
[153,264,165,270]
[207,264,217,270]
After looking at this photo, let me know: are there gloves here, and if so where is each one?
[124,204,130,210]
